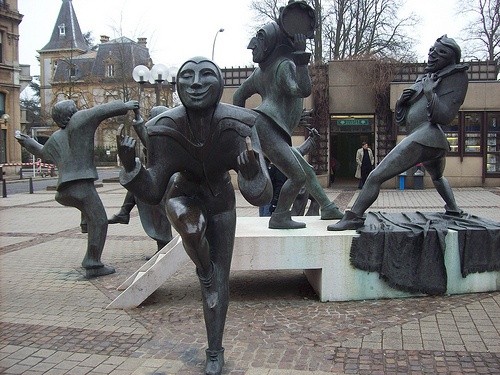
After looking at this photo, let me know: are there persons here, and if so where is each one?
[106,99,170,260]
[327,34,469,231]
[14,100,140,278]
[116,56,273,375]
[232,20,345,230]
[354,142,374,190]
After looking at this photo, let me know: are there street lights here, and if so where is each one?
[211,28,225,62]
[131,63,180,107]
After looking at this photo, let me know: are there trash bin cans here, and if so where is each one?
[413,176,423,190]
[397,175,407,190]
